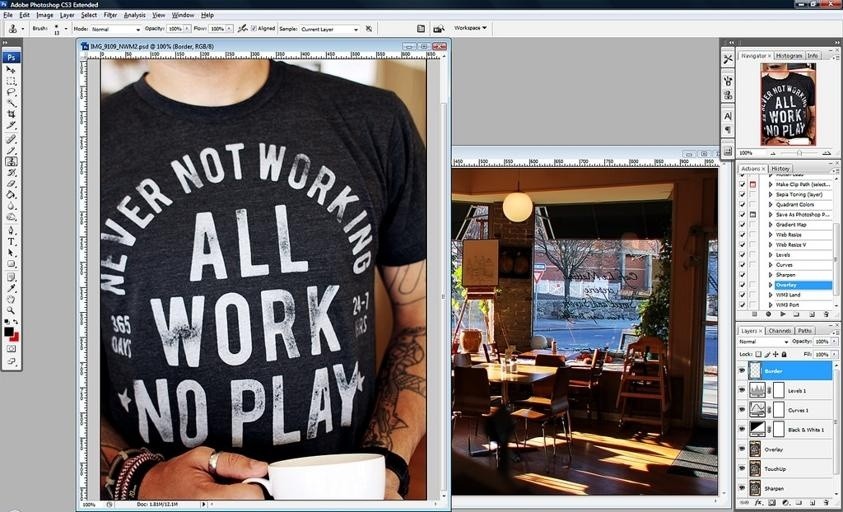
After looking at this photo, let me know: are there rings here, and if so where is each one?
[207,449,223,476]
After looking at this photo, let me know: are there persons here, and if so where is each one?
[760,63,816,146]
[101,59,427,501]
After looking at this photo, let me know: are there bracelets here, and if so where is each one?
[761,136,771,146]
[806,135,814,144]
[353,445,411,498]
[101,443,164,501]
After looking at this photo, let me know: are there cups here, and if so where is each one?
[242,453,386,501]
[787,137,809,146]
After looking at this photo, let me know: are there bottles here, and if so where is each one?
[551,338,557,354]
[500,358,518,374]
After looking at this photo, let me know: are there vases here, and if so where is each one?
[462,329,482,353]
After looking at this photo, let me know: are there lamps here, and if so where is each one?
[502,169,533,223]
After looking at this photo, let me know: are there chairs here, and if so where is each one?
[451,342,609,475]
